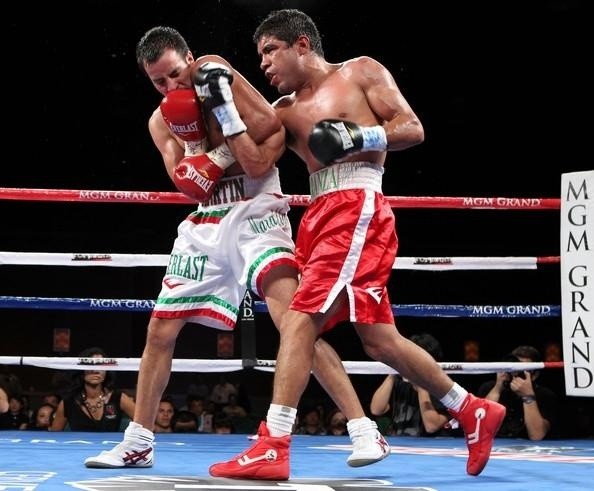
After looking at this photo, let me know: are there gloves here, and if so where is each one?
[161,88,205,158]
[172,142,234,203]
[194,61,248,137]
[307,119,386,167]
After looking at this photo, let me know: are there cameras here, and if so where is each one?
[504,353,526,386]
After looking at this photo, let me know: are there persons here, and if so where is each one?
[81,26,392,469]
[0,346,594,442]
[192,8,506,483]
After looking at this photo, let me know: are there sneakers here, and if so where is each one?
[346,415,391,467]
[209,421,291,481]
[447,392,507,476]
[85,420,155,468]
